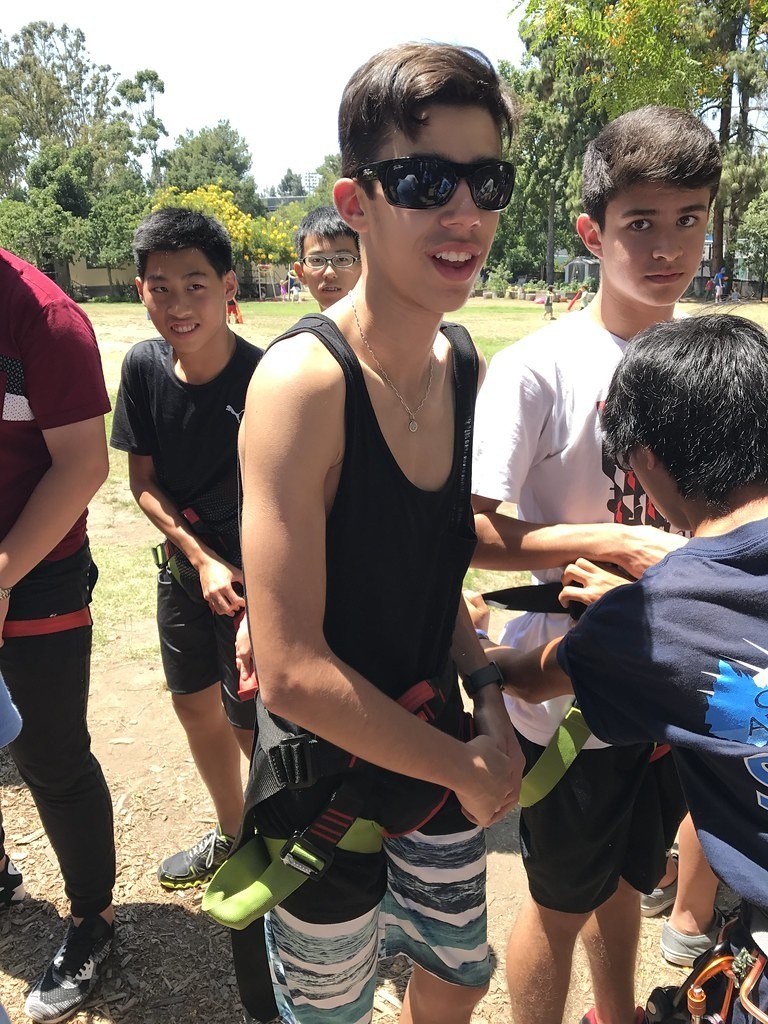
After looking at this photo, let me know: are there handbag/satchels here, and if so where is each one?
[646,917,768,1024]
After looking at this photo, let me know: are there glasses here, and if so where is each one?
[299,253,359,269]
[614,437,642,472]
[350,156,517,212]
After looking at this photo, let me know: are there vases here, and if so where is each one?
[475,287,596,303]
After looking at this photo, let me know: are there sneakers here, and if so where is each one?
[24,914,115,1024]
[157,824,258,889]
[0,854,25,906]
[660,909,737,967]
[640,852,678,918]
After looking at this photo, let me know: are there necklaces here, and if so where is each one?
[349,289,434,432]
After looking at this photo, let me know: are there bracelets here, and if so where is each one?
[0,587,11,600]
[474,628,489,640]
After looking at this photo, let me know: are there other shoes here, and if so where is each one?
[579,1007,650,1024]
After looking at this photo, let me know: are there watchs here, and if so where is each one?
[462,661,506,698]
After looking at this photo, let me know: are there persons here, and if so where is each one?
[113,205,265,892]
[235,42,527,1024]
[0,252,116,1024]
[541,285,557,321]
[576,284,589,311]
[469,104,721,1024]
[294,206,362,313]
[705,268,742,303]
[463,311,768,965]
[227,268,302,325]
